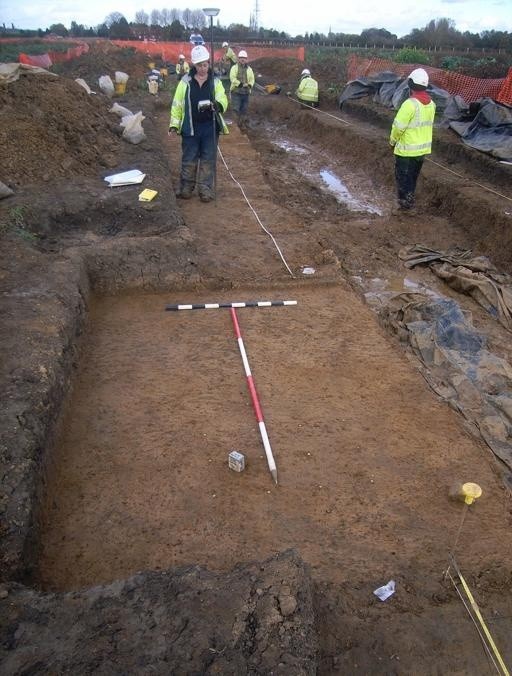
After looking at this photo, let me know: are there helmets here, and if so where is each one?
[189,44,210,65]
[300,68,311,76]
[237,49,248,59]
[407,67,429,88]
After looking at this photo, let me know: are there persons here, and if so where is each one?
[174,53,190,81]
[165,45,229,202]
[388,68,437,211]
[230,48,257,128]
[219,41,237,69]
[295,67,319,111]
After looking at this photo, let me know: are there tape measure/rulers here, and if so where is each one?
[444,482,482,580]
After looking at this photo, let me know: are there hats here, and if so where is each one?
[222,42,228,49]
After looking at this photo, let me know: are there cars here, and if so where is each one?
[189,33,206,46]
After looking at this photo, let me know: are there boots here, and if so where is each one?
[175,161,198,198]
[197,158,215,203]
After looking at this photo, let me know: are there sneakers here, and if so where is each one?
[390,199,418,217]
